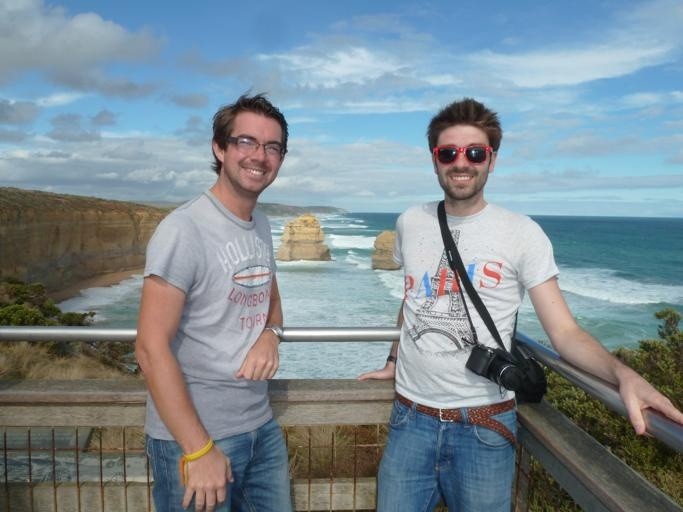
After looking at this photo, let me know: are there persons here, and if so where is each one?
[130,96,293,511]
[354,95,683,511]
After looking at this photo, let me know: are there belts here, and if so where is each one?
[394,391,518,447]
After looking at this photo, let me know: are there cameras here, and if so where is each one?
[465,345,524,391]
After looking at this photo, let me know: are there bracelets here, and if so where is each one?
[384,354,397,363]
[180,437,214,463]
[264,325,284,343]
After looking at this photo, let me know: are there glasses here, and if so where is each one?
[223,133,286,157]
[430,143,494,167]
